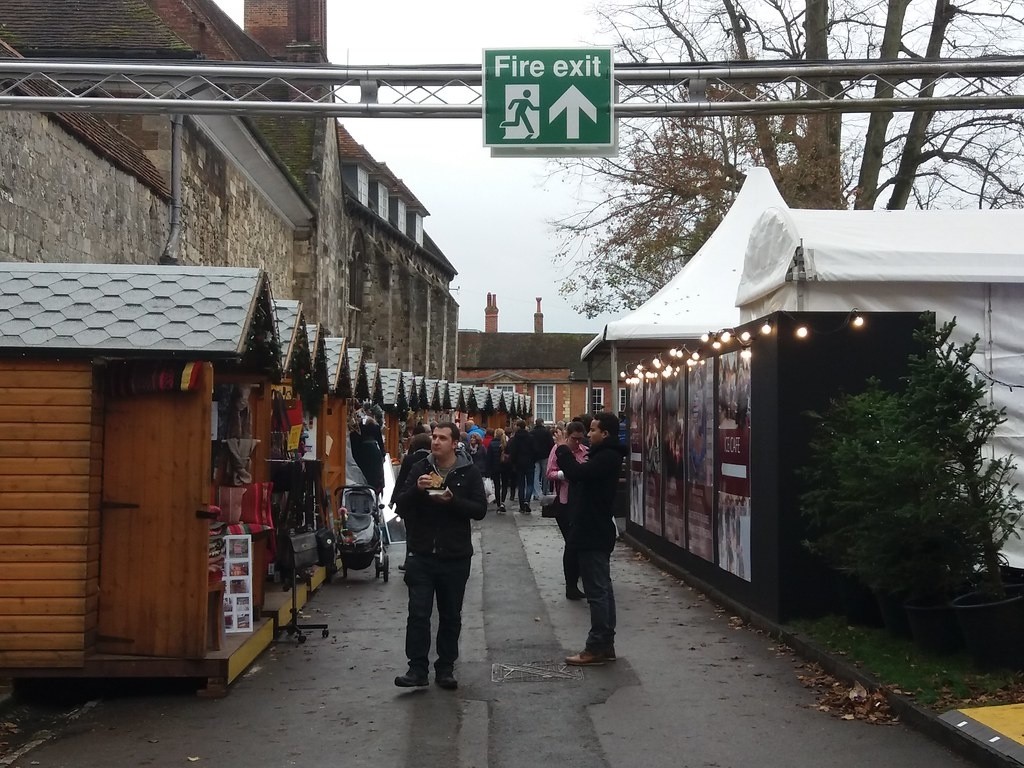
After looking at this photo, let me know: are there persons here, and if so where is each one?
[482,428,495,448]
[394,422,487,690]
[466,432,488,478]
[465,420,485,443]
[403,414,592,488]
[488,429,507,514]
[389,433,431,572]
[717,356,750,430]
[503,419,537,514]
[552,411,624,665]
[717,493,746,572]
[545,422,591,600]
[530,417,553,501]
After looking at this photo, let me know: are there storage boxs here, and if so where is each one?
[218,485,247,523]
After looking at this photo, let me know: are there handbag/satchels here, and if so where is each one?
[500,450,511,463]
[540,495,560,518]
[271,462,304,496]
[484,477,492,498]
[287,526,335,570]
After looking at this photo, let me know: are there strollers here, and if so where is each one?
[329,485,390,581]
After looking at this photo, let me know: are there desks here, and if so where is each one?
[207,579,228,651]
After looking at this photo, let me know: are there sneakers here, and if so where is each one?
[435,671,457,689]
[394,669,430,687]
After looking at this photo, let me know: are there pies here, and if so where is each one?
[430,472,443,488]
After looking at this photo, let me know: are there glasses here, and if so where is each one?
[570,435,584,442]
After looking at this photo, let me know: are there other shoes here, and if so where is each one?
[524,502,532,512]
[496,509,501,514]
[566,650,617,665]
[500,502,506,512]
[519,509,525,513]
[566,590,586,599]
